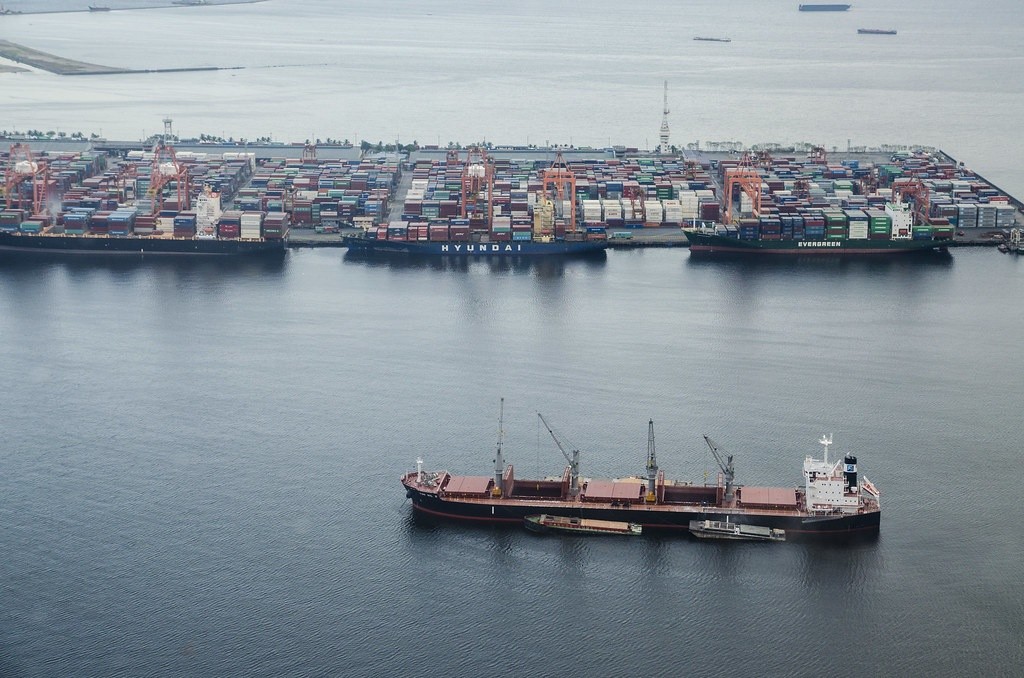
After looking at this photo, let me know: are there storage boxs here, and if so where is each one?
[0,141,1019,239]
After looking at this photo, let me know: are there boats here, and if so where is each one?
[689,520,786,542]
[523,513,642,536]
[998,244,1008,252]
[681,227,957,258]
[340,232,610,256]
[0,231,291,257]
[401,398,882,533]
